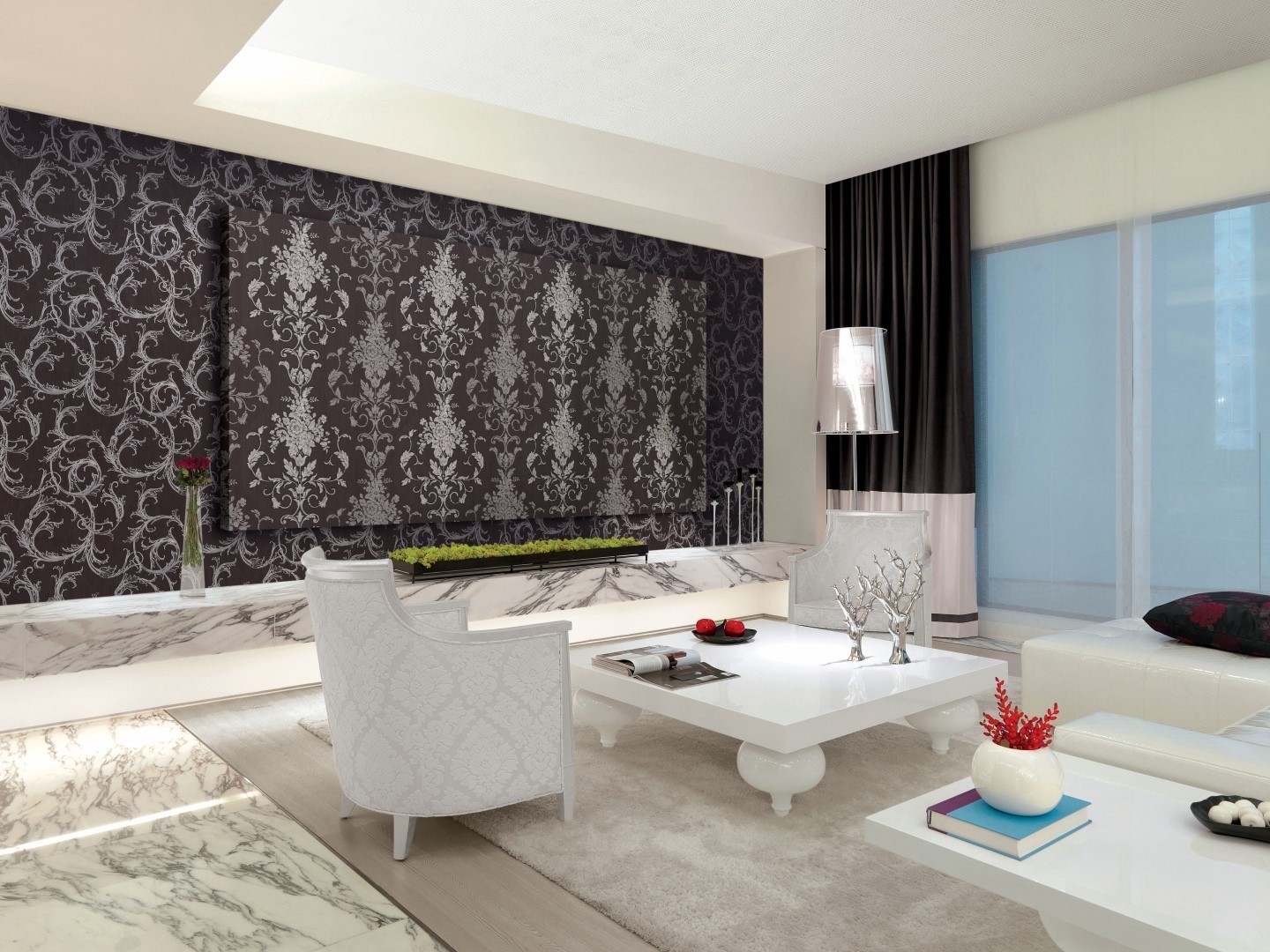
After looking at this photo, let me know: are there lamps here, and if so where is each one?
[812,327,899,511]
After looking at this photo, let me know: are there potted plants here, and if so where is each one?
[389,537,649,584]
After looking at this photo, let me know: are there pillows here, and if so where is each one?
[1142,591,1270,659]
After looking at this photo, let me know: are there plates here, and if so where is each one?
[692,618,757,643]
[1191,796,1270,843]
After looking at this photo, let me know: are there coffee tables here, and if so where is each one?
[569,618,1008,816]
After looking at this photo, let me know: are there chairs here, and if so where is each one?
[788,508,933,648]
[301,546,575,860]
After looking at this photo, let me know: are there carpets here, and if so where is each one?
[298,710,1067,952]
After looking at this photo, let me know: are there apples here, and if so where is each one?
[723,620,745,637]
[696,619,717,637]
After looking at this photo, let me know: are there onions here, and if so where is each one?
[1208,799,1270,827]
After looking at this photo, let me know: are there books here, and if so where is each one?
[927,789,1092,861]
[591,645,741,691]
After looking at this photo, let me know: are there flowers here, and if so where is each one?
[173,456,211,568]
[978,677,1060,751]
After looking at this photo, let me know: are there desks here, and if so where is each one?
[864,749,1270,952]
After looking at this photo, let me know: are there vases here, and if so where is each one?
[971,739,1065,816]
[179,485,206,597]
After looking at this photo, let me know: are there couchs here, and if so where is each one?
[1021,616,1270,803]
